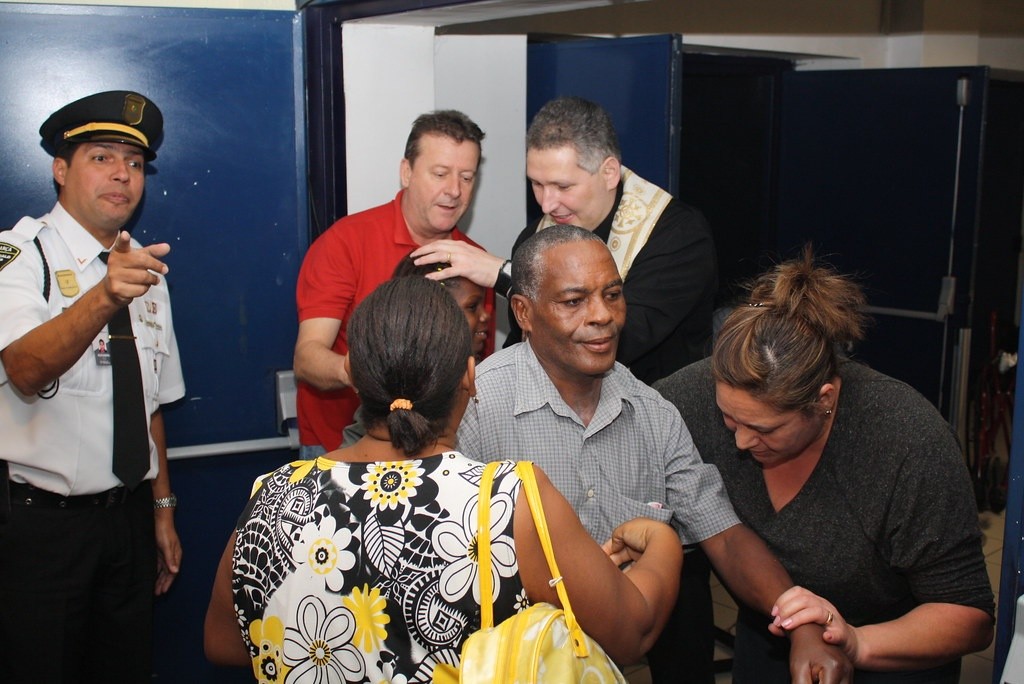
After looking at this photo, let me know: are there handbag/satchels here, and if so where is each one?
[458,460,629,684]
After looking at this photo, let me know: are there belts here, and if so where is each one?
[9,480,130,510]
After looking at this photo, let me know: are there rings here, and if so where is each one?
[447,252,451,263]
[823,610,833,625]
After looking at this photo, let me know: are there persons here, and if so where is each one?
[0,90,185,684]
[294,110,497,461]
[98,338,107,353]
[336,251,490,450]
[447,224,857,684]
[411,95,718,387]
[203,275,684,684]
[648,255,997,684]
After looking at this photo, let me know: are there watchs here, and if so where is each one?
[491,259,513,299]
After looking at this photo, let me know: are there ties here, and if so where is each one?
[98,251,151,493]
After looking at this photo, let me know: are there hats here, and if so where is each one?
[40,90,164,161]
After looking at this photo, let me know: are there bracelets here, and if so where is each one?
[154,493,177,508]
